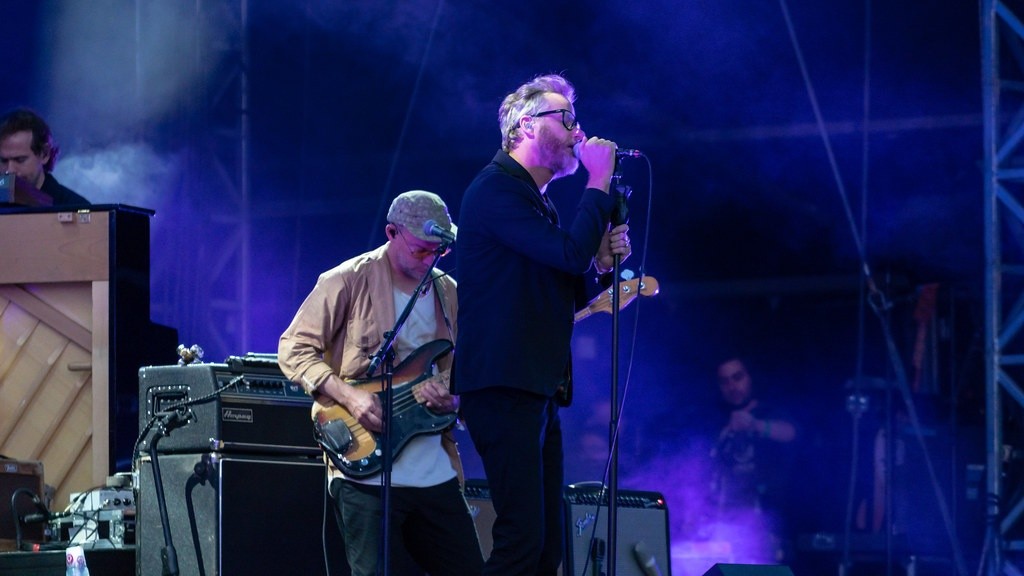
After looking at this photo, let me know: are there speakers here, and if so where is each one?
[702,563,794,576]
[563,481,672,576]
[136,453,354,576]
[0,458,45,552]
[464,484,498,561]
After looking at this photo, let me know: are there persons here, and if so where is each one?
[718,358,798,442]
[451,76,632,576]
[0,109,88,205]
[277,190,485,575]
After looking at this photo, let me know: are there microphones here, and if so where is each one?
[573,142,644,158]
[635,541,662,576]
[423,220,456,242]
[589,537,605,576]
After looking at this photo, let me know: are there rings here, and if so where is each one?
[439,404,442,407]
[624,235,628,240]
[625,239,630,246]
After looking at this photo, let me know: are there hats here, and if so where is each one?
[387,191,458,242]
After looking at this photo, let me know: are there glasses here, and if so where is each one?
[394,226,450,259]
[515,109,582,131]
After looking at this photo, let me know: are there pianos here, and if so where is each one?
[1,204,176,515]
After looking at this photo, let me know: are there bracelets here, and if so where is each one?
[765,420,770,434]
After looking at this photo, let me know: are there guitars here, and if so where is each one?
[310,266,660,482]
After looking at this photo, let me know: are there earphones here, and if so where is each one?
[525,122,533,129]
[389,228,396,239]
